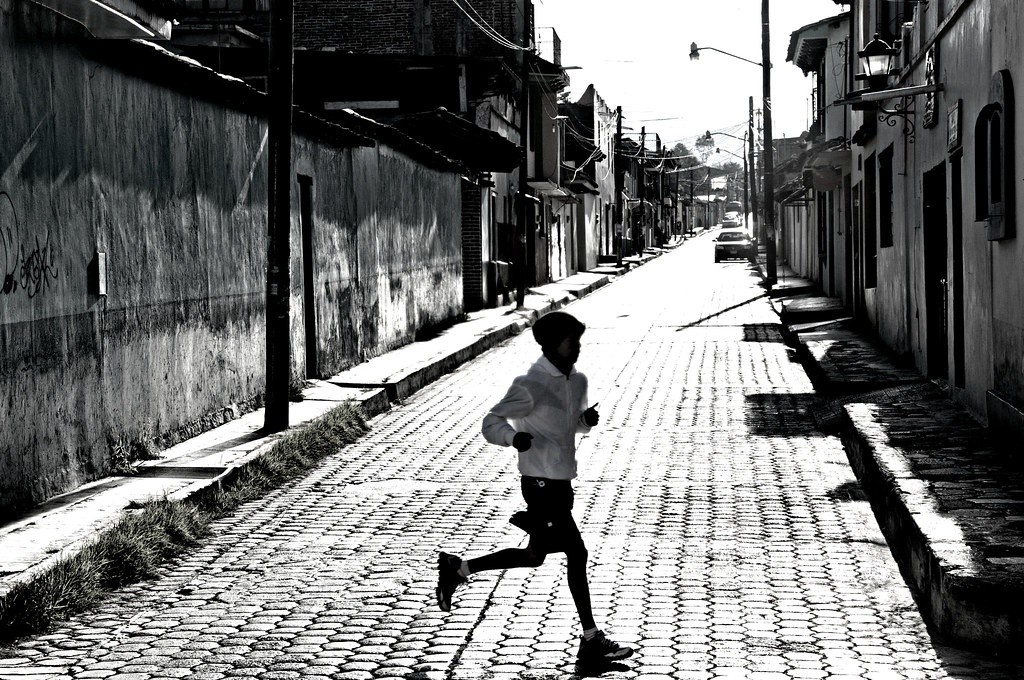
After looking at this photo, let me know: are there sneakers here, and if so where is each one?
[436,552,468,612]
[577,630,634,660]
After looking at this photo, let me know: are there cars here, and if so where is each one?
[711,230,758,263]
[721,210,740,228]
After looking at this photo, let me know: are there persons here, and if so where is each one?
[659,228,665,248]
[509,234,527,311]
[638,234,645,257]
[437,312,634,661]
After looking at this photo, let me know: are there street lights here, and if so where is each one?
[690,0,778,285]
[706,95,759,257]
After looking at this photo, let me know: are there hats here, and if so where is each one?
[532,311,583,346]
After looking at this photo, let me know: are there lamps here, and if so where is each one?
[857,33,916,144]
